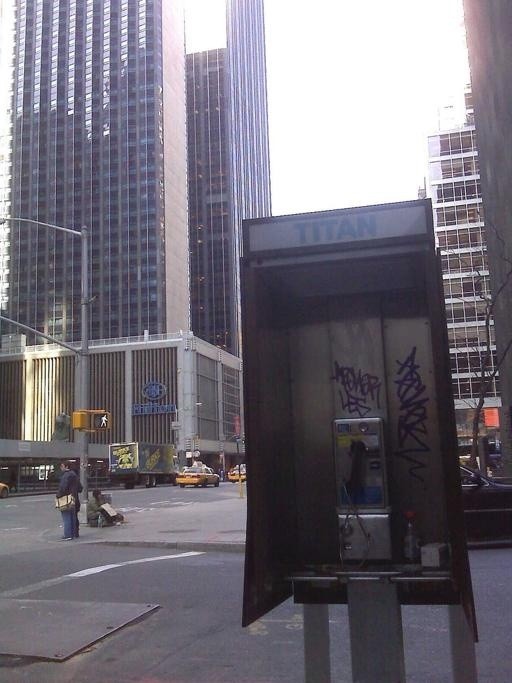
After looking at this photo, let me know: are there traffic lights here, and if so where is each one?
[72,412,111,432]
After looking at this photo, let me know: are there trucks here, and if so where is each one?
[109,442,179,489]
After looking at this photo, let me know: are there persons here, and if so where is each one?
[86,489,104,520]
[56,461,83,541]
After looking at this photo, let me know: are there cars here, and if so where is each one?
[176,461,247,487]
[456,435,502,460]
[0,483,9,498]
[458,464,512,550]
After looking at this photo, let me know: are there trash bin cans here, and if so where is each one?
[102,494,111,503]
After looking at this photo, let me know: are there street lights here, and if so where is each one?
[0,216,91,528]
[172,402,202,444]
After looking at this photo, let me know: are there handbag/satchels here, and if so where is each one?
[55,494,76,512]
[100,502,118,521]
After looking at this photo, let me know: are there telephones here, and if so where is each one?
[334,418,389,514]
